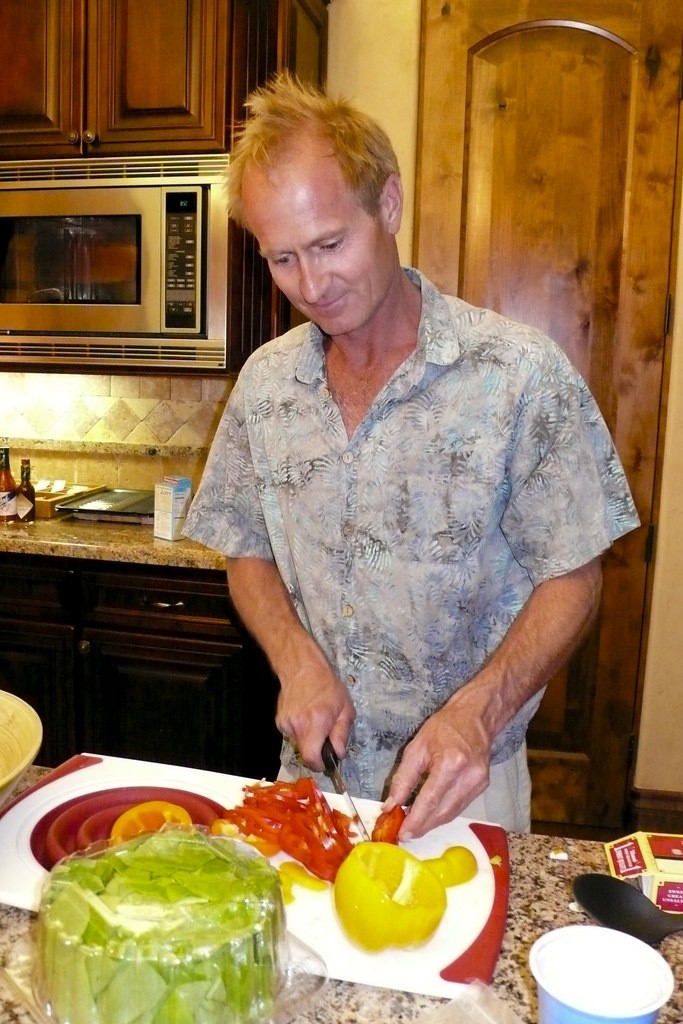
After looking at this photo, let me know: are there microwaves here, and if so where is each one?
[1,154,267,379]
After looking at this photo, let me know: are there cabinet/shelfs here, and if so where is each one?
[0,515,284,784]
[0,0,329,161]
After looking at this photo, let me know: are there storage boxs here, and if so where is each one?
[16,480,108,519]
[165,474,192,515]
[153,481,186,542]
[603,831,683,914]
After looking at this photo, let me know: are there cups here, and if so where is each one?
[528,925,675,1024]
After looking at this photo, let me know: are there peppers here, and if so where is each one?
[334,841,478,951]
[110,801,333,904]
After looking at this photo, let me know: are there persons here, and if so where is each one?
[178,69,642,846]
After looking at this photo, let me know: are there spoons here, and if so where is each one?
[572,874,682,947]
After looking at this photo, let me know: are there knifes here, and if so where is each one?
[322,736,372,841]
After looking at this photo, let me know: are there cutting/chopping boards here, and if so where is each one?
[0,753,512,1000]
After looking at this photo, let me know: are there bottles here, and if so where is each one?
[0,447,17,523]
[16,458,36,522]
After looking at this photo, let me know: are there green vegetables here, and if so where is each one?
[35,830,287,1023]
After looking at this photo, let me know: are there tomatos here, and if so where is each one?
[222,775,406,885]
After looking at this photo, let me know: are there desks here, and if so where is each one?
[0,765,683,1024]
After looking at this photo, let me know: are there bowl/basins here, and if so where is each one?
[0,688,43,806]
